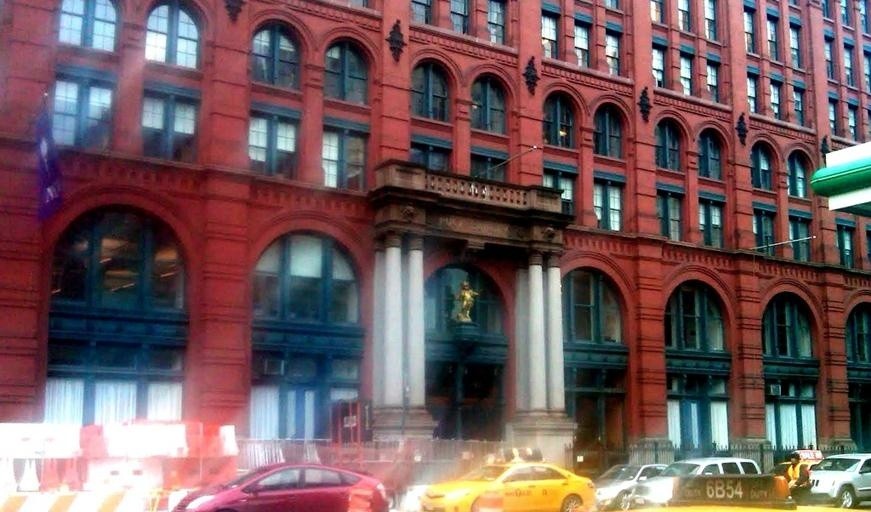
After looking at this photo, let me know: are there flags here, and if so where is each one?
[35,102,65,221]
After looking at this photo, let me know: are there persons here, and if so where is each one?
[456,281,480,320]
[785,453,813,505]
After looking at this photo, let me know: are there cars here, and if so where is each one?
[416,451,598,512]
[168,458,395,512]
[588,446,871,512]
